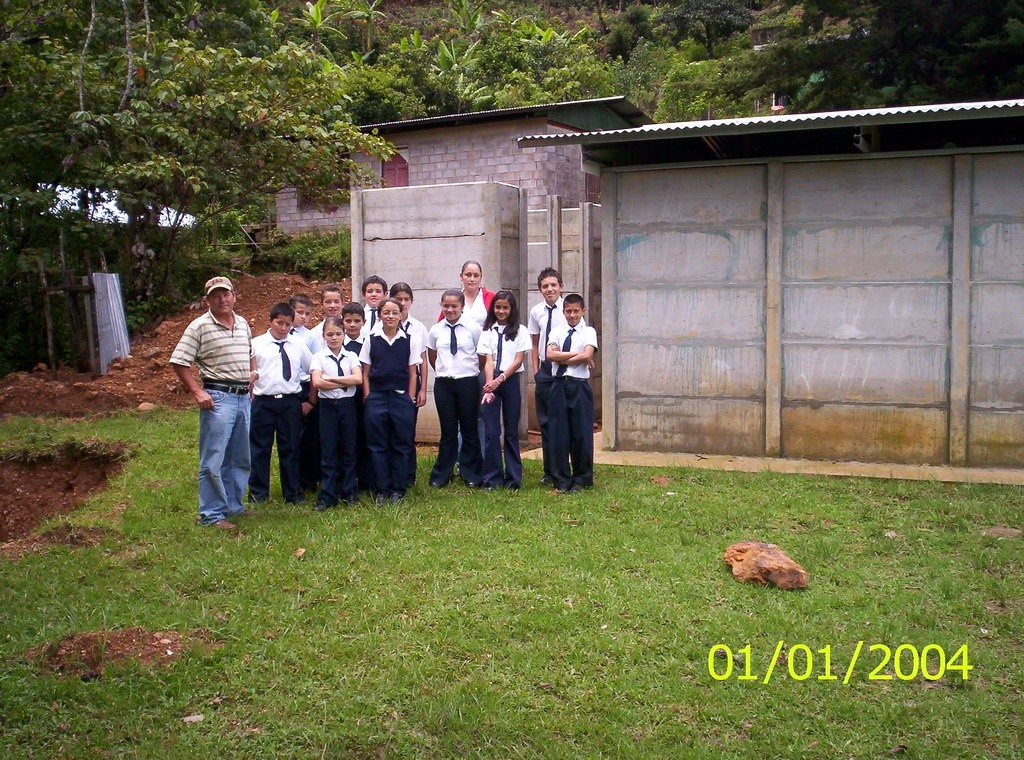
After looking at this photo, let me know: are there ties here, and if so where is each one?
[370,309,377,330]
[494,327,506,377]
[274,342,291,382]
[545,305,557,360]
[330,356,347,392]
[556,328,576,377]
[446,324,457,356]
[289,328,295,335]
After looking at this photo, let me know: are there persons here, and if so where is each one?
[309,316,363,510]
[171,275,259,531]
[250,302,317,503]
[546,294,598,492]
[357,299,422,504]
[438,260,497,472]
[339,302,377,492]
[529,267,585,484]
[428,290,487,488]
[389,282,429,489]
[288,294,323,493]
[477,290,527,491]
[305,275,389,359]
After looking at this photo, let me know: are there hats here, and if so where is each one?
[205,276,233,295]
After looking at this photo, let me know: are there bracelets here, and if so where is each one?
[251,370,259,380]
[500,373,506,382]
[495,379,501,386]
[308,400,316,407]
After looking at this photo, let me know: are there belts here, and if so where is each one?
[204,383,249,395]
[554,376,587,382]
[255,393,300,398]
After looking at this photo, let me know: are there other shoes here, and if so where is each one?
[240,510,262,517]
[541,474,553,485]
[196,516,238,531]
[484,484,501,492]
[556,487,573,494]
[504,483,520,491]
[571,483,589,493]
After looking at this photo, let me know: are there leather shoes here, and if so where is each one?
[312,503,331,511]
[374,491,389,506]
[390,492,403,505]
[465,479,481,488]
[429,479,443,490]
[344,498,368,508]
